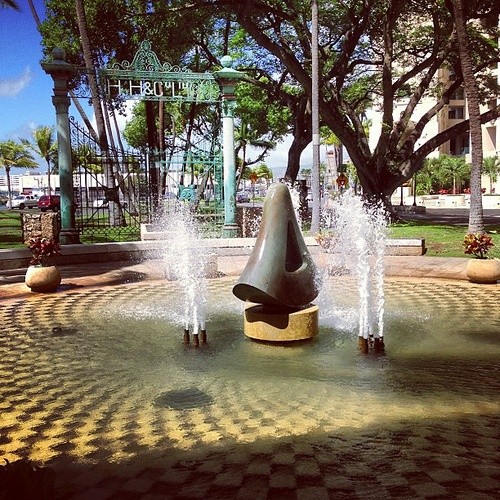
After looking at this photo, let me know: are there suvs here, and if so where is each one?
[38,196,78,212]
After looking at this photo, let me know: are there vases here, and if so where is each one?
[318,252,346,275]
[25,265,61,293]
[467,258,500,283]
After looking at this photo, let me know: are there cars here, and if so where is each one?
[94,196,129,208]
[0,197,9,205]
[6,195,37,210]
[236,195,250,203]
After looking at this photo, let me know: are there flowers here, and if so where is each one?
[315,230,342,251]
[24,236,64,262]
[464,231,493,255]
